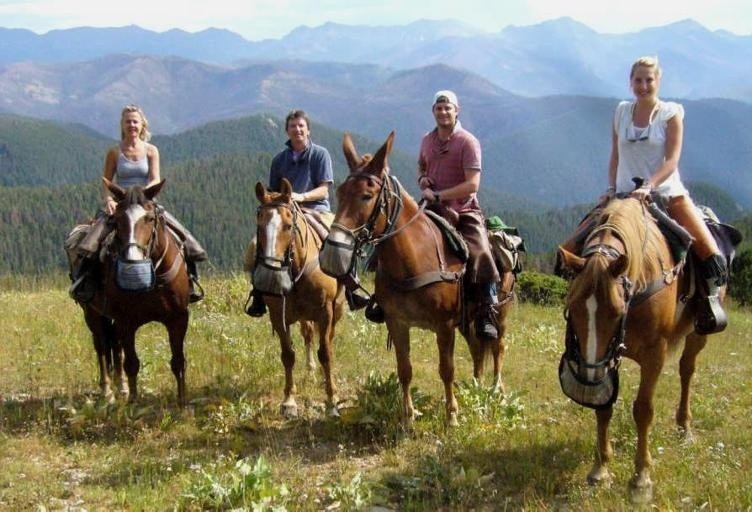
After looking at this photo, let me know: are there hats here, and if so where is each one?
[431,89,460,110]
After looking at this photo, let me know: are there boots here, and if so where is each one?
[186,261,200,299]
[477,304,499,340]
[371,305,386,323]
[248,281,266,314]
[344,271,370,311]
[75,256,93,299]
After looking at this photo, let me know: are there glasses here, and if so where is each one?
[625,119,651,141]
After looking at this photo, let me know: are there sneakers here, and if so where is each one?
[699,298,715,332]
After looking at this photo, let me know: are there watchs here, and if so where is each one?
[432,191,441,203]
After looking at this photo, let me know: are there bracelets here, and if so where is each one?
[606,186,617,192]
[639,182,654,191]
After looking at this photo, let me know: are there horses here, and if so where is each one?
[253,177,347,423]
[557,194,728,508]
[83,175,191,415]
[318,129,517,430]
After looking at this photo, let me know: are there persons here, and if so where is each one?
[598,54,729,333]
[373,89,501,340]
[248,109,370,317]
[68,104,198,304]
